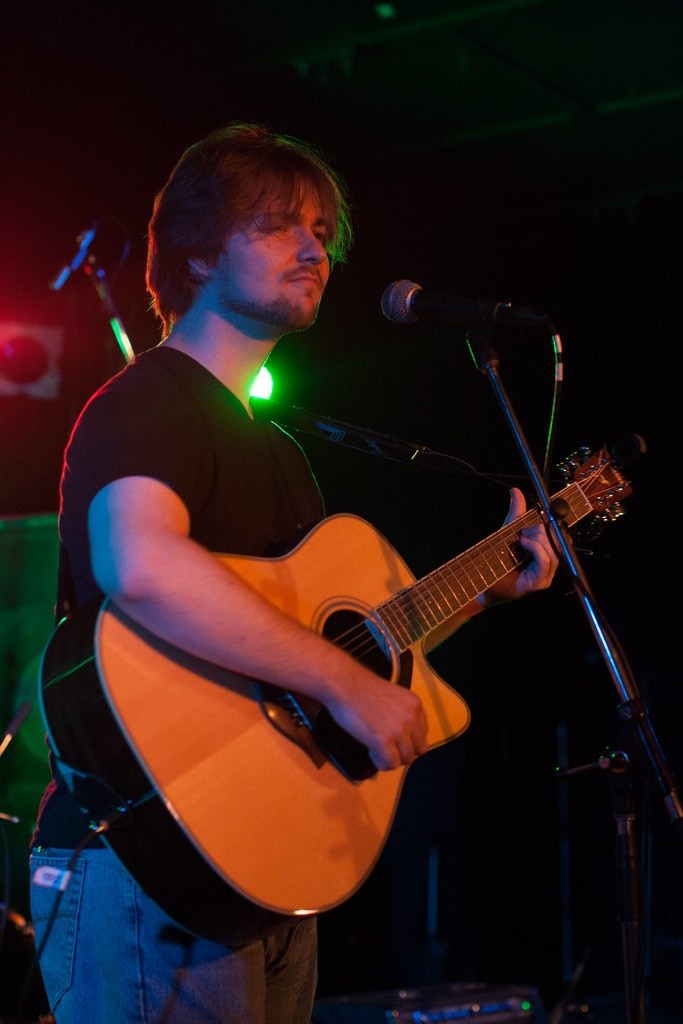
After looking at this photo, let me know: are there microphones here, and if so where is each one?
[380,280,550,323]
[48,220,97,291]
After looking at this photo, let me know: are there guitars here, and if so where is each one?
[35,426,653,952]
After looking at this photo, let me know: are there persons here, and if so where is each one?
[28,125,565,1024]
